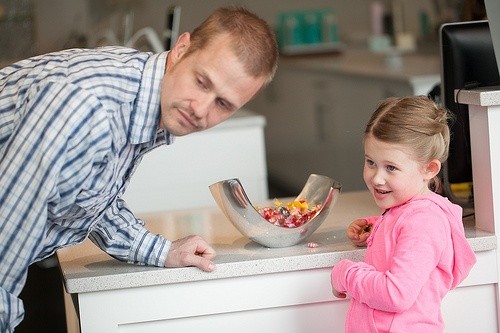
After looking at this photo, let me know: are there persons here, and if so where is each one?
[0,5,280,333]
[329,95,476,333]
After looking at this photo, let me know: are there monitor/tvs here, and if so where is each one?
[162,6,181,53]
[440,20,500,185]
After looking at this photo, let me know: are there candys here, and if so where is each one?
[253,197,322,227]
[306,241,320,247]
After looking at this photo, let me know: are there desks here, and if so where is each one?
[50,187,500,333]
